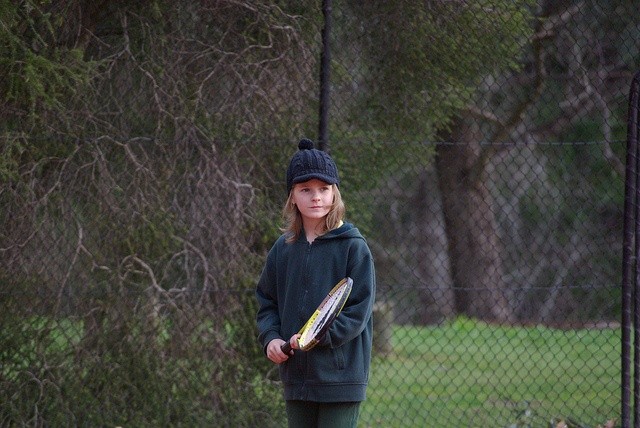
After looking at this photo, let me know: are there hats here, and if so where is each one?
[287,138,340,194]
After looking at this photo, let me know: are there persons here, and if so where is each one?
[256,140,377,428]
